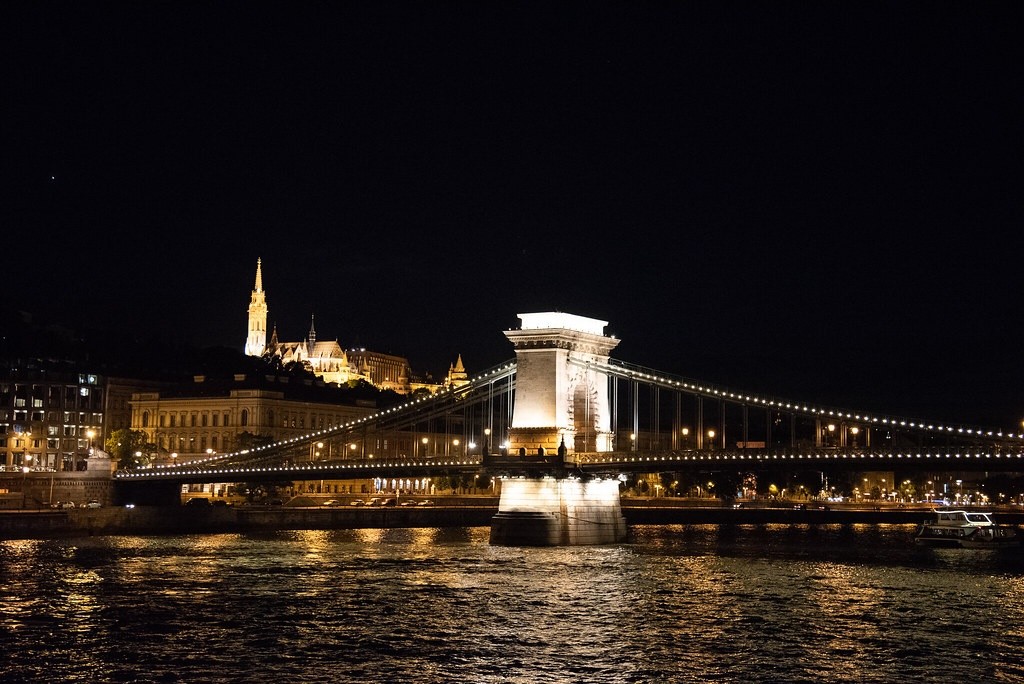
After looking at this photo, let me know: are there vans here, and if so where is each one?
[365,498,382,507]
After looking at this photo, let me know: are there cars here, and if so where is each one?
[400,500,417,506]
[418,499,435,506]
[351,500,365,507]
[734,503,745,508]
[80,499,102,509]
[50,500,76,510]
[185,495,235,507]
[323,499,340,507]
[381,498,396,507]
[795,503,807,510]
[819,506,829,511]
[264,498,283,506]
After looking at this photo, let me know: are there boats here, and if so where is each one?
[914,507,1024,550]
